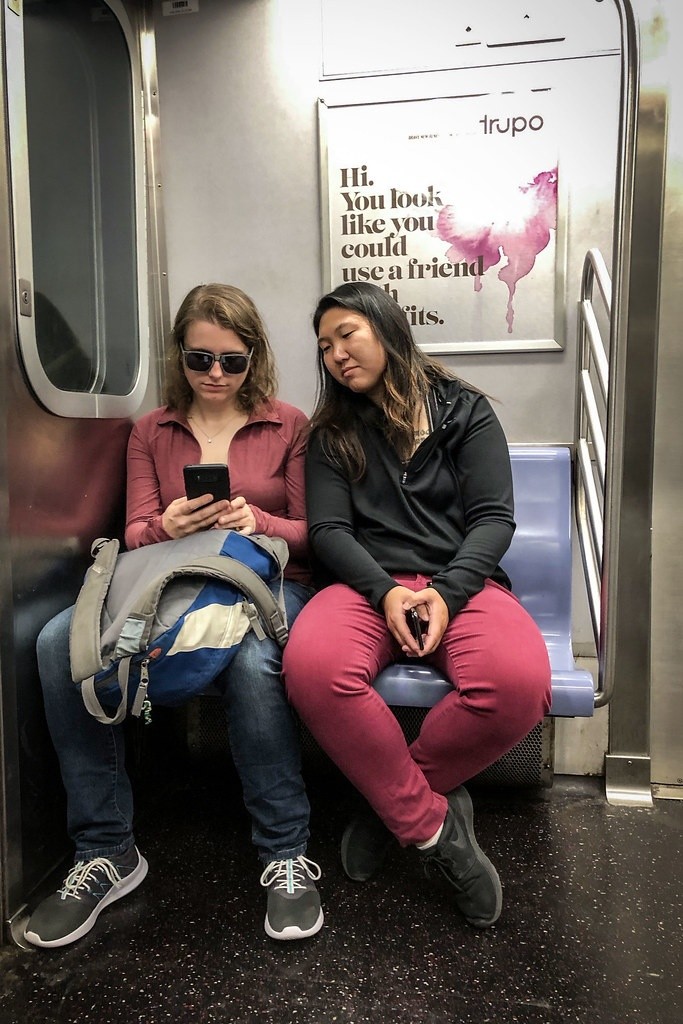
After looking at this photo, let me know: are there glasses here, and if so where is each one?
[174,338,258,377]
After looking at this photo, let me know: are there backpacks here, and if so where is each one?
[70,534,290,725]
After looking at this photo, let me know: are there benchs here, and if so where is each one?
[173,443,594,753]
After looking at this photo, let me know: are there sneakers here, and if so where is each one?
[23,844,147,948]
[340,806,391,883]
[414,786,501,927]
[264,853,328,942]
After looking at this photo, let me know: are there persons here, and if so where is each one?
[282,282,552,928]
[22,284,324,947]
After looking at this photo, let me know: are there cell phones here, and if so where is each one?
[405,607,424,651]
[184,464,230,512]
